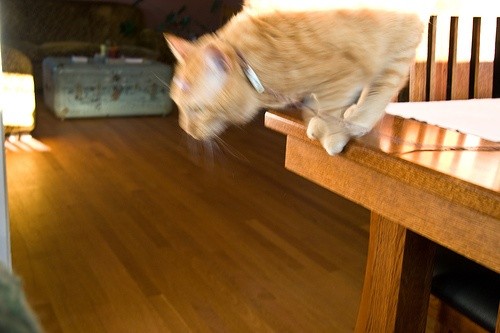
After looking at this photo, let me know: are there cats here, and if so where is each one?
[162,0,426,156]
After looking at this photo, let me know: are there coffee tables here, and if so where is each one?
[41,55,173,121]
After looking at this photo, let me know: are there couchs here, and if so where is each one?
[2,3,160,145]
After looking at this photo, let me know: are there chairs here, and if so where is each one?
[397,15,500,333]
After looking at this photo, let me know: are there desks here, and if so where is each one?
[264,98,500,333]
